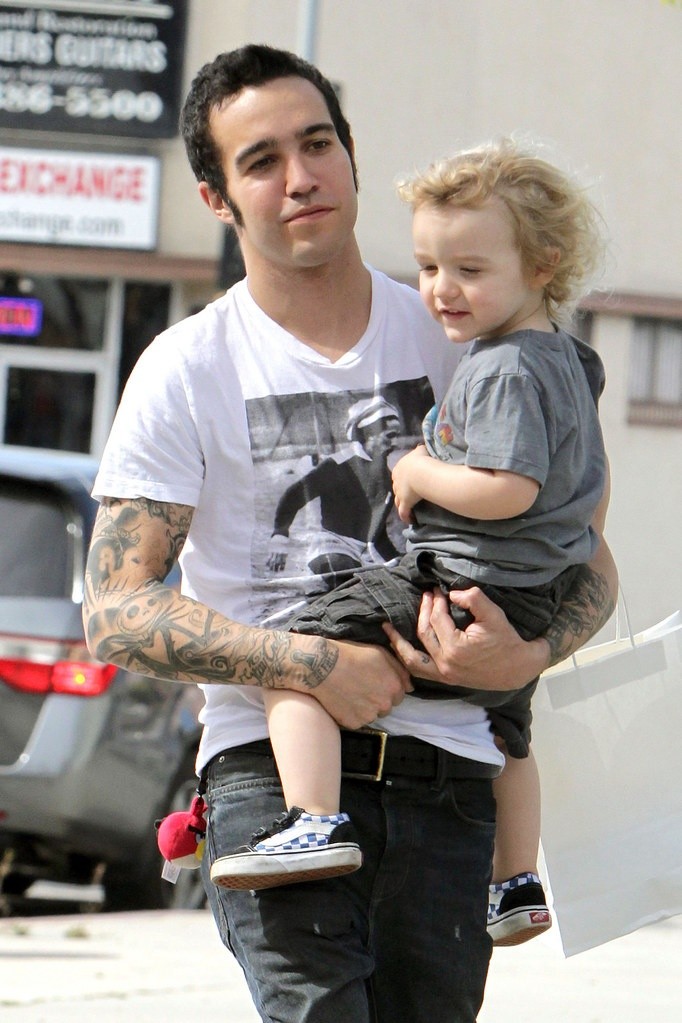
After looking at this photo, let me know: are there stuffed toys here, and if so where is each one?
[154,796,208,868]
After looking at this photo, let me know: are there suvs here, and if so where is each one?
[0,444,211,910]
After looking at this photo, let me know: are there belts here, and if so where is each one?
[216,728,502,782]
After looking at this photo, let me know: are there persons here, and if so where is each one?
[82,45,619,1022]
[212,144,607,943]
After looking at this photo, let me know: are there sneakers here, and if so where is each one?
[210,805,362,891]
[484,873,552,946]
